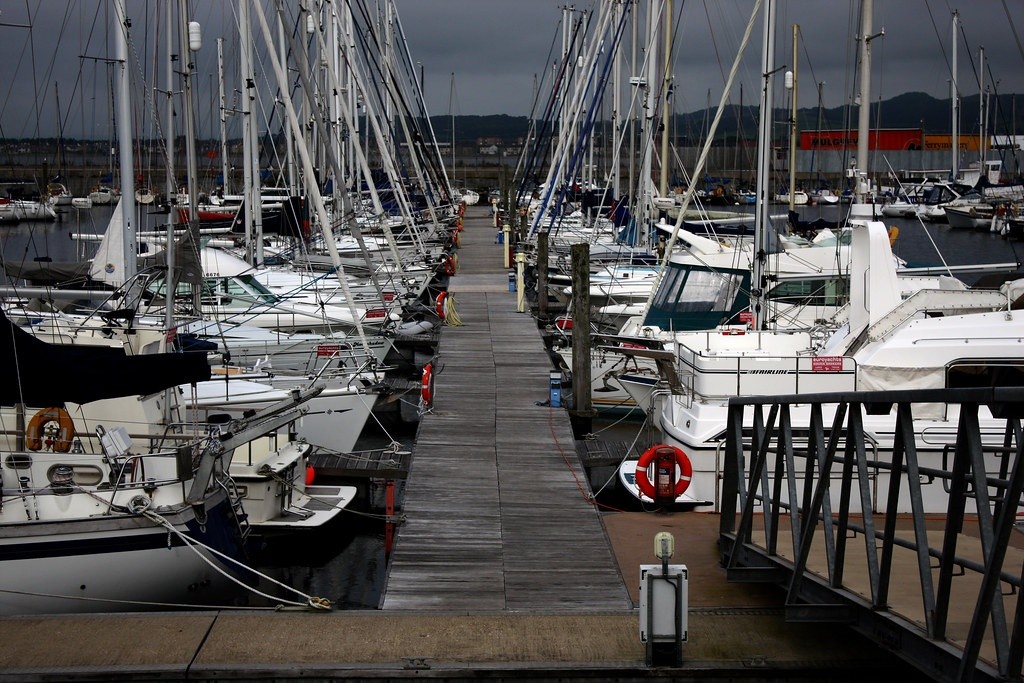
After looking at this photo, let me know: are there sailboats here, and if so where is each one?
[1,0,483,632]
[489,0,1024,516]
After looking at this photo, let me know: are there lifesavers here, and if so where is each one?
[421,363,432,402]
[450,206,467,247]
[635,444,693,499]
[26,407,74,452]
[437,291,447,319]
[445,256,455,276]
[888,226,899,246]
